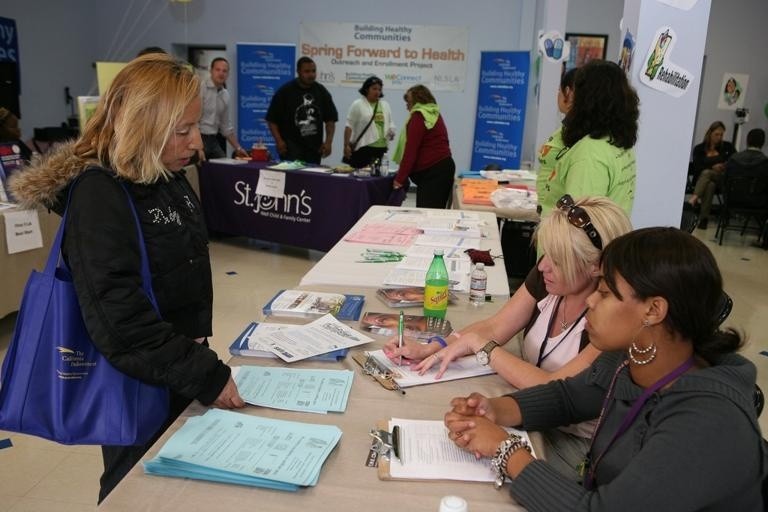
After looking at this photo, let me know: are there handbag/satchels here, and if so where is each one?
[340,98,381,161]
[0,169,171,449]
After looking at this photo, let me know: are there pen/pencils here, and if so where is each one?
[397,311,404,367]
[387,375,406,395]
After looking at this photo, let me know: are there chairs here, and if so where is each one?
[715,176,768,248]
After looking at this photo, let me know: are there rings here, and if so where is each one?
[433,352,441,361]
[455,431,463,438]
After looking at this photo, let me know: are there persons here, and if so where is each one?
[722,127,768,222]
[537,67,583,210]
[536,58,640,268]
[265,57,338,165]
[199,58,249,167]
[393,84,456,209]
[345,76,395,168]
[8,52,244,506]
[684,121,737,228]
[445,227,768,512]
[382,194,636,481]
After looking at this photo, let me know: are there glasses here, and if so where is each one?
[555,194,602,253]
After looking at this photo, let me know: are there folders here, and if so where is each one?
[352,342,498,390]
[369,418,544,485]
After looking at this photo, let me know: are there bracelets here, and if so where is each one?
[489,433,532,491]
[427,336,449,349]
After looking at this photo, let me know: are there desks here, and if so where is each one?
[200,157,409,254]
[454,178,540,276]
[92,205,538,512]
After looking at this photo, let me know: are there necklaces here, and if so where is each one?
[556,295,583,333]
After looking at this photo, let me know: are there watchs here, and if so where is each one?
[476,339,501,368]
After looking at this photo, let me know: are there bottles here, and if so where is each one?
[424,248,449,319]
[469,263,487,306]
[381,153,389,176]
